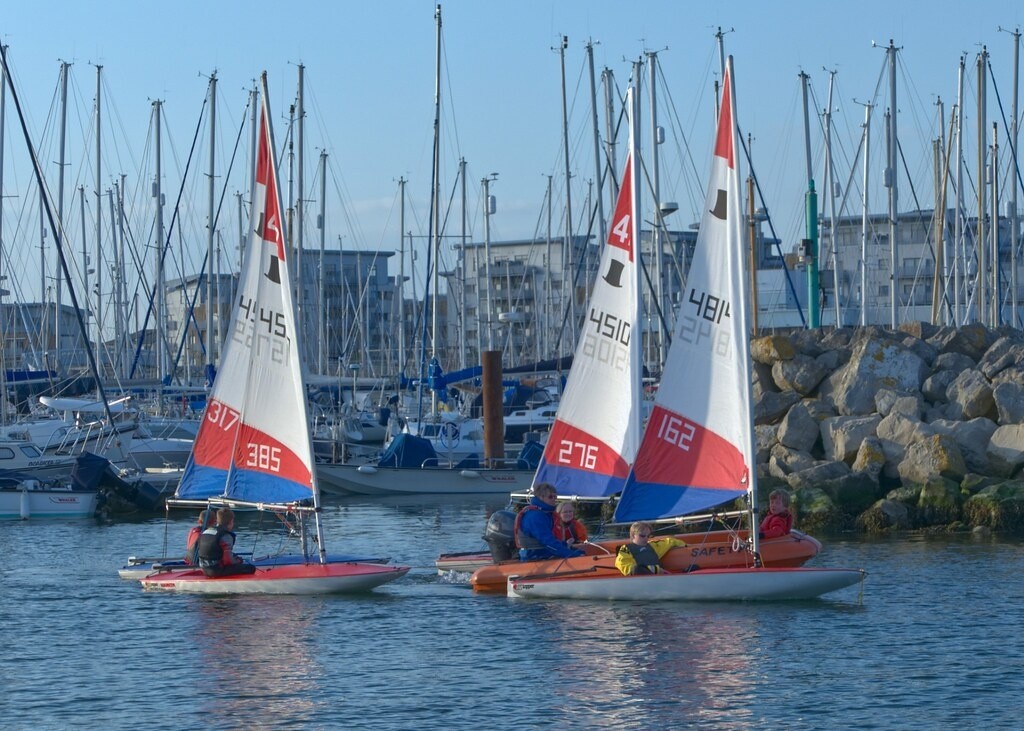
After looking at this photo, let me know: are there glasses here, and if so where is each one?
[633,531,653,537]
[543,495,557,499]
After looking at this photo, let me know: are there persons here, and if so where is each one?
[515,483,586,563]
[759,490,793,540]
[613,521,690,577]
[193,510,256,581]
[184,509,213,558]
[555,501,584,545]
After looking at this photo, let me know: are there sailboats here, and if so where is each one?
[118,71,394,581]
[437,85,823,594]
[140,70,410,595]
[0,0,657,520]
[508,55,868,602]
